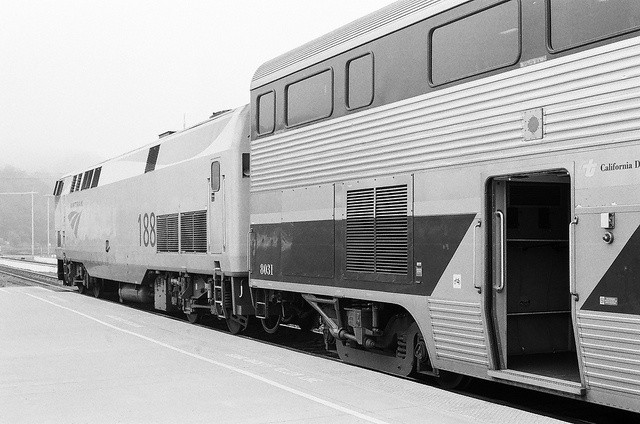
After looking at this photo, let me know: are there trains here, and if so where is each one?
[53,0,640,413]
